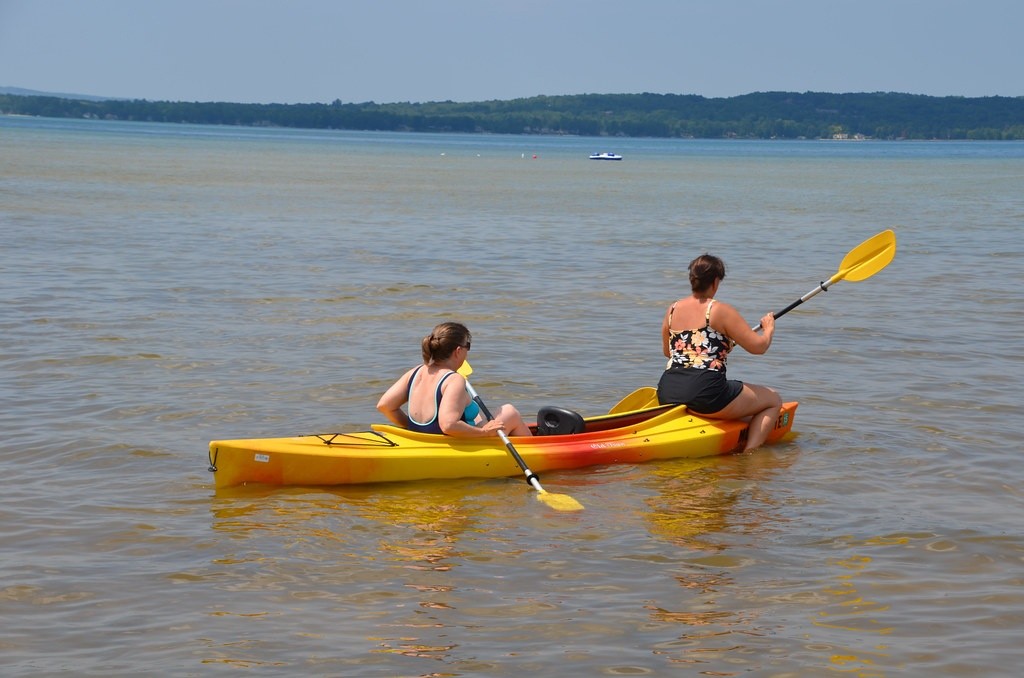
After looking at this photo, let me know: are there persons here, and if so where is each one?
[376,323,532,439]
[656,255,782,452]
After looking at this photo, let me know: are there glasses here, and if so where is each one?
[462,342,470,351]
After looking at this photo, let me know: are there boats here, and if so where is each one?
[208,386,798,489]
[590,153,622,161]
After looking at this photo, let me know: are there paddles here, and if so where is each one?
[457,360,586,511]
[608,229,895,415]
